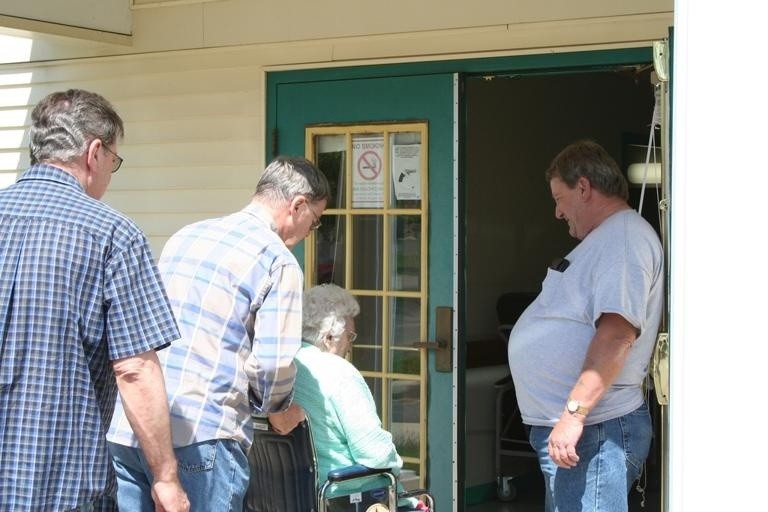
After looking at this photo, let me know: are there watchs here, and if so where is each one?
[565,397,591,416]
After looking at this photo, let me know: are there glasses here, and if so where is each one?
[101,141,123,173]
[344,329,359,344]
[306,205,323,231]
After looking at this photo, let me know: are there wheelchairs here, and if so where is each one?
[243,409,435,512]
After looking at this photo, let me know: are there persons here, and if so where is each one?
[284,283,422,512]
[0,88,192,512]
[507,141,664,512]
[107,154,331,512]
